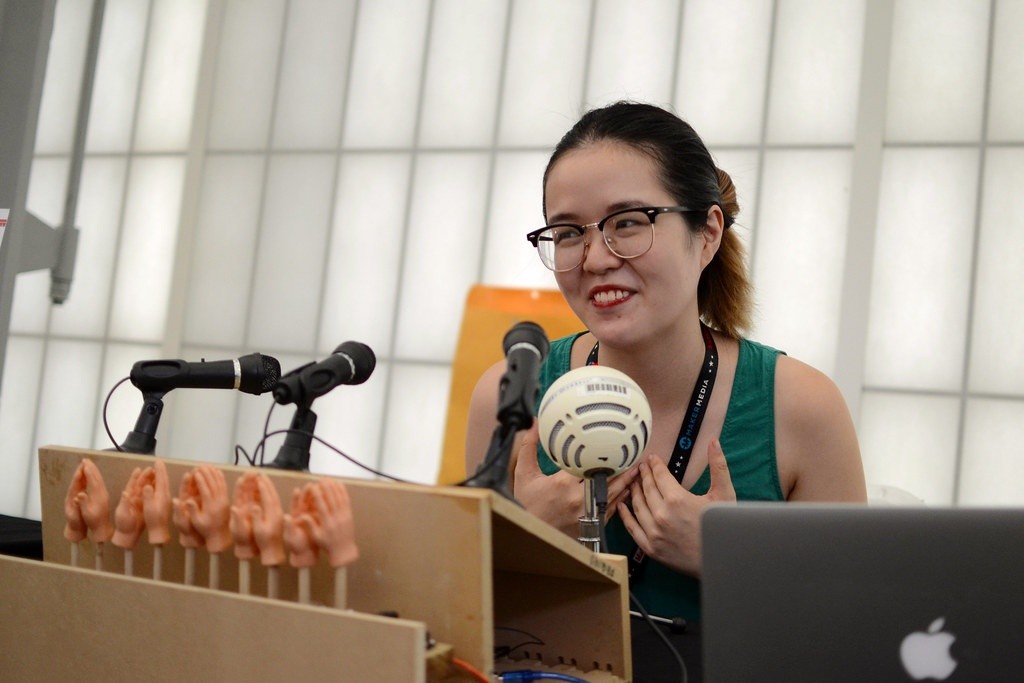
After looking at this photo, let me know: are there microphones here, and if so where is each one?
[130,353,281,395]
[502,320,547,428]
[538,365,653,516]
[273,340,376,405]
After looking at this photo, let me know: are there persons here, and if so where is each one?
[463,101,868,681]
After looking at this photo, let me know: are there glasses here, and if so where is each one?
[526,205,695,272]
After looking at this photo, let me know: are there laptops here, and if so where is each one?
[700,501,1024,683]
